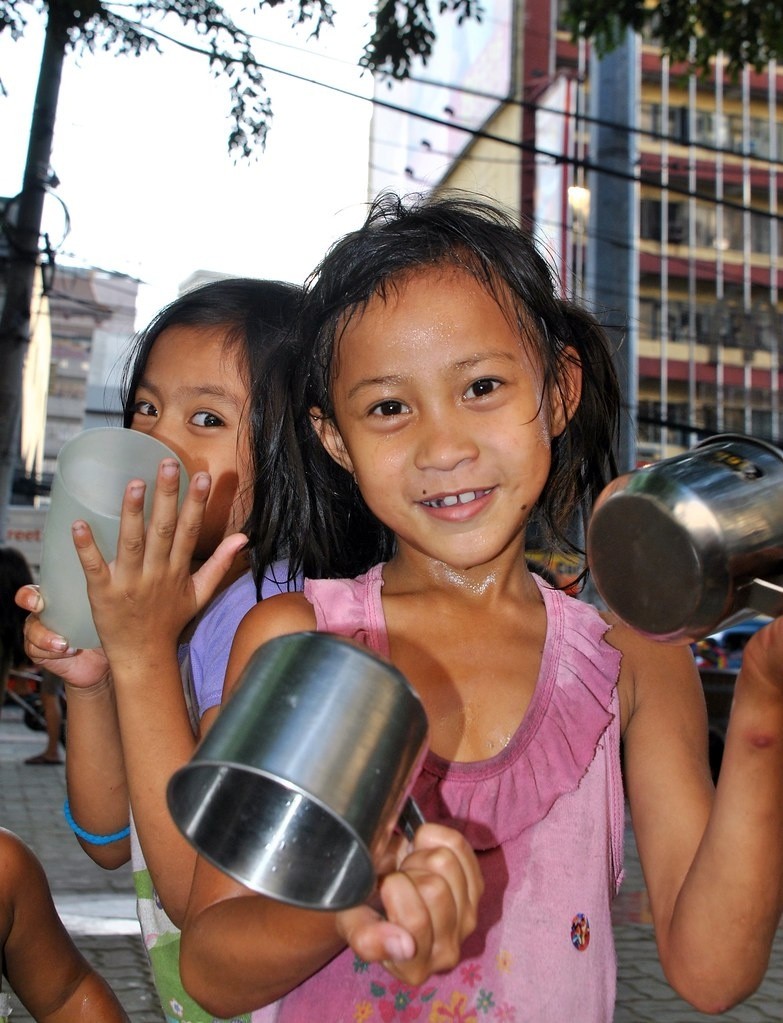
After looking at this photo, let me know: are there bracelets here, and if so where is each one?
[63,797,131,846]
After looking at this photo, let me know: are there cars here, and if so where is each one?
[691,616,774,786]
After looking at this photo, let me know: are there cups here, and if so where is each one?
[167,631,432,911]
[38,427,189,649]
[587,432,783,646]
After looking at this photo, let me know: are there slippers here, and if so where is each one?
[26,754,63,766]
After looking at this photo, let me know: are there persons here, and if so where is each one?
[177,188,777,1023]
[14,277,396,1023]
[0,828,129,1023]
